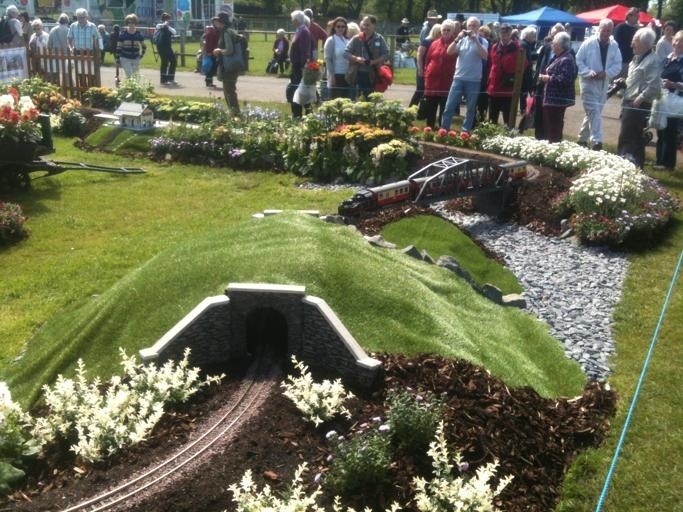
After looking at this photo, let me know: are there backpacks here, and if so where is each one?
[0,16,17,43]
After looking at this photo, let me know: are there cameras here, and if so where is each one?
[464,30,472,36]
[606,78,626,100]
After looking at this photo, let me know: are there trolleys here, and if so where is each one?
[0,112,149,193]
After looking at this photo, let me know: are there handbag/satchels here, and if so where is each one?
[649,85,683,130]
[222,31,248,74]
[375,65,393,91]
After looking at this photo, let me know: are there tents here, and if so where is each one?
[576,4,653,27]
[499,4,591,53]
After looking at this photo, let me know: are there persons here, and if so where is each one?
[409,10,682,170]
[153,11,245,112]
[117,14,146,77]
[0,5,121,72]
[272,8,409,118]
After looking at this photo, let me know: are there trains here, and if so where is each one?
[336,161,527,215]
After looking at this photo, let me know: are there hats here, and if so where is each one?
[427,9,442,20]
[401,18,410,24]
